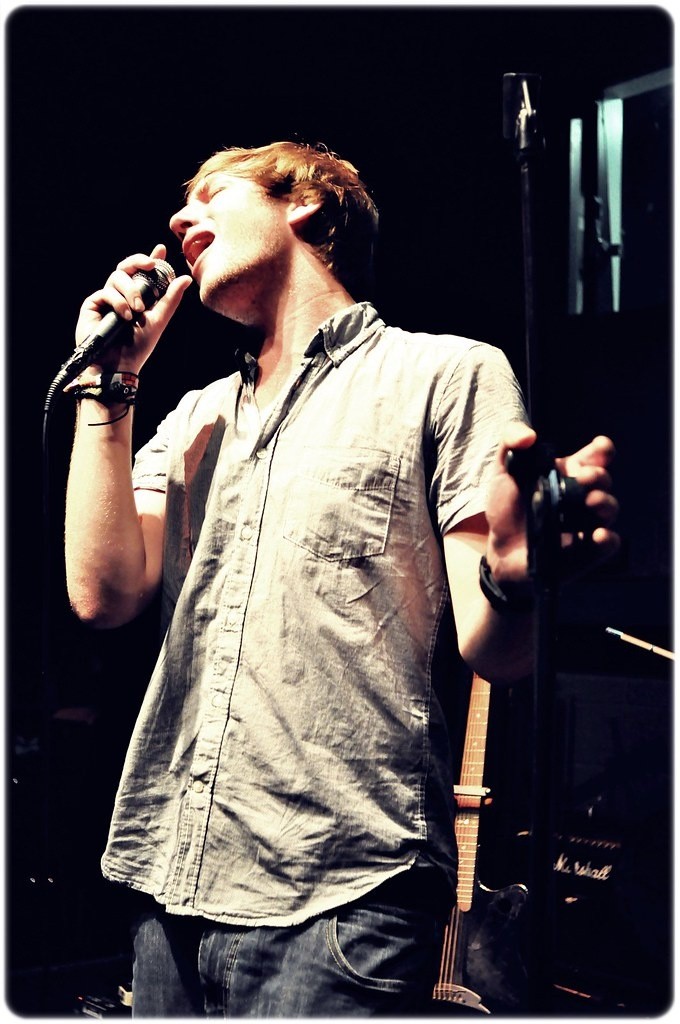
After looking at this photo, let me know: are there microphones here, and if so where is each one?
[44,259,175,413]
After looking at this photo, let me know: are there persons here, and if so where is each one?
[60,138,626,1018]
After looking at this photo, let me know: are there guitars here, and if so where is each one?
[406,657,538,1016]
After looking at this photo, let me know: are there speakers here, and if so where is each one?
[539,831,655,1012]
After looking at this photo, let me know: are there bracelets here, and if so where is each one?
[58,366,144,431]
[477,554,538,614]
